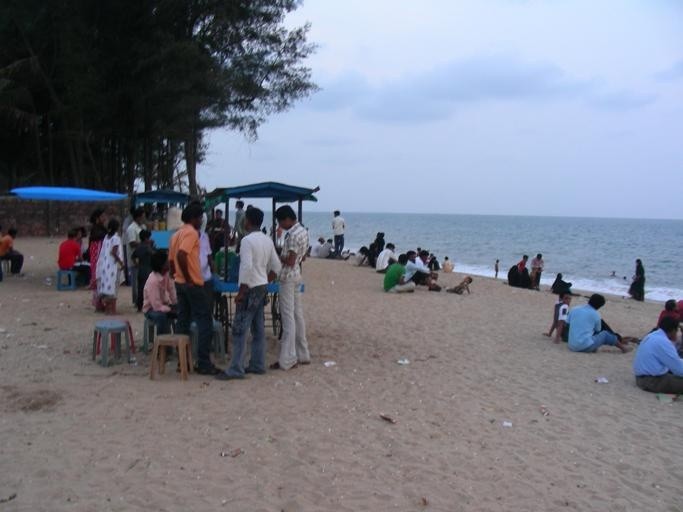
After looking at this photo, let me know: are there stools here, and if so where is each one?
[54,269,226,382]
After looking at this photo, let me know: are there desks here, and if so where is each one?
[211,279,305,355]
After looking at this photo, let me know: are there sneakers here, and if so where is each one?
[429,283,441,291]
[196,363,219,375]
[217,371,244,380]
[177,362,198,372]
[269,362,298,369]
[244,367,266,374]
[147,342,154,353]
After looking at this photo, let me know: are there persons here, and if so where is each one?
[627,258,644,301]
[0,228,23,276]
[564,293,634,354]
[622,276,626,279]
[609,271,615,276]
[313,210,473,295]
[493,259,499,278]
[54,194,310,378]
[541,288,628,346]
[508,252,573,295]
[635,298,682,395]
